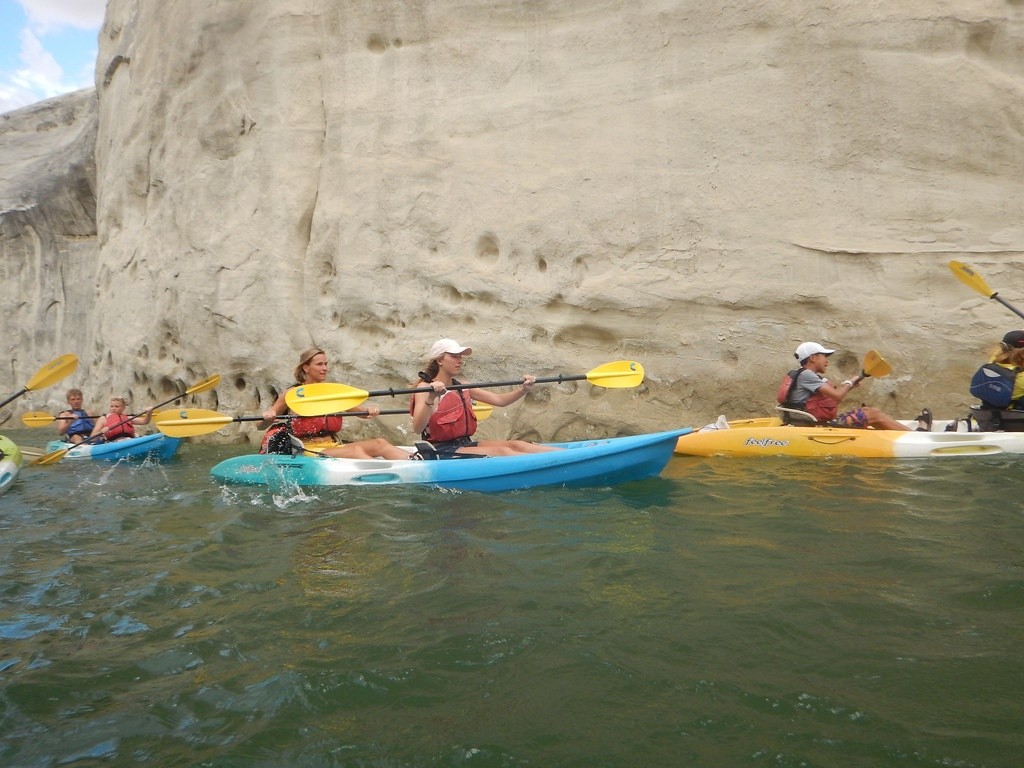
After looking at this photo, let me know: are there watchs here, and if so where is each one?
[841,380,852,389]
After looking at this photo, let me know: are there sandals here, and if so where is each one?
[915,408,932,432]
[946,418,958,432]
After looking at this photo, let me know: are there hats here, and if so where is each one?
[795,342,835,362]
[1002,330,1024,348]
[430,339,472,358]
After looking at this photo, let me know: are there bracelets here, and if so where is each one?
[425,401,434,407]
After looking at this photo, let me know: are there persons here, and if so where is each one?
[775,341,958,432]
[58,388,106,445]
[256,348,412,461]
[89,397,154,446]
[992,330,1024,432]
[410,338,566,458]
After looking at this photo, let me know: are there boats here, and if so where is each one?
[209,427,698,493]
[44,433,184,465]
[0,435,22,494]
[672,405,1024,465]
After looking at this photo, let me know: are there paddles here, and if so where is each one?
[33,374,221,464]
[19,446,47,461]
[950,260,1024,319]
[21,411,160,428]
[0,354,78,408]
[155,401,493,440]
[285,360,646,418]
[850,349,892,390]
